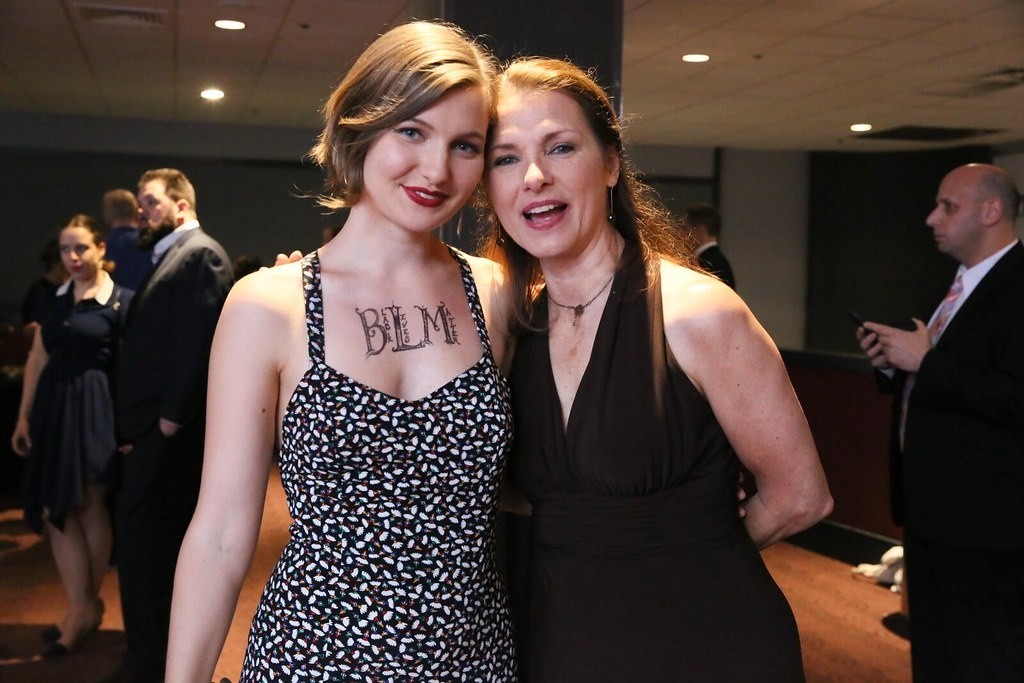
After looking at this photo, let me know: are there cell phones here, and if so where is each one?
[845,312,883,356]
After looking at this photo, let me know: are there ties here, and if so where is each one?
[899,275,967,443]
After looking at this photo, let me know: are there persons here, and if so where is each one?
[323,223,342,246]
[10,167,235,683]
[855,163,1024,683]
[685,203,734,291]
[233,254,258,283]
[164,21,541,683]
[258,57,835,683]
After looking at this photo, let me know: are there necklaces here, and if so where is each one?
[548,275,614,327]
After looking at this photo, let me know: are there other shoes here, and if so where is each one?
[38,622,74,645]
[31,596,106,661]
